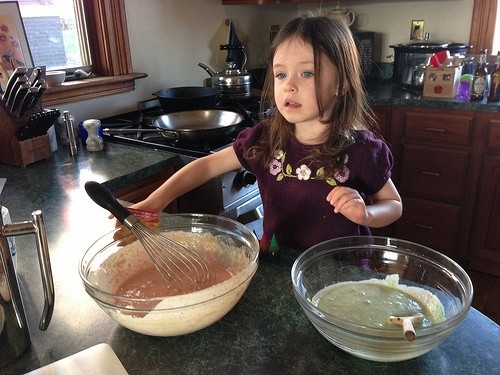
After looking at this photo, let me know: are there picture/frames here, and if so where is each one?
[0,1,35,100]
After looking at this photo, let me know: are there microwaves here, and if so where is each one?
[270,24,374,84]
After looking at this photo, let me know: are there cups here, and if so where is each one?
[0,205,17,278]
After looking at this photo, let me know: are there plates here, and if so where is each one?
[22,342,129,375]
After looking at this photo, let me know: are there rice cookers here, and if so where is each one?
[388,32,472,89]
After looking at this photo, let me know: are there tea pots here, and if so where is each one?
[198,44,251,96]
[323,6,355,26]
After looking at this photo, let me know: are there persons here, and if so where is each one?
[109,14,402,270]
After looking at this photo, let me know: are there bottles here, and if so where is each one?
[487,51,500,101]
[470,48,487,100]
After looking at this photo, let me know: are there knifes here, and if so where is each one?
[2,66,60,140]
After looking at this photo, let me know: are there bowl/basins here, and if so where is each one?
[291,236,474,362]
[45,70,66,86]
[78,214,260,336]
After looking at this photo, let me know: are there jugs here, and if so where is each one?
[0,209,55,367]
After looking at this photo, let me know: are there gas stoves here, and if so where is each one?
[89,83,275,220]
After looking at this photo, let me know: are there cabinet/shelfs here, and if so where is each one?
[111,168,177,215]
[370,105,500,280]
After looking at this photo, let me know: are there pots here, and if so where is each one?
[103,110,244,145]
[152,86,223,109]
